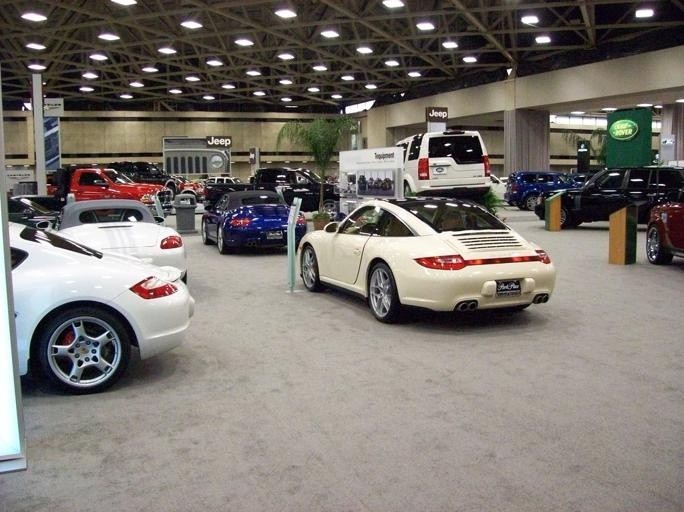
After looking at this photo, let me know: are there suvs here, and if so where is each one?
[533,165,683,229]
[641,184,684,265]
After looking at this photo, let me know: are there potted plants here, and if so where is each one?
[274,114,359,231]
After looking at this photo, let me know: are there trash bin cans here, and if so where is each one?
[173,194,199,234]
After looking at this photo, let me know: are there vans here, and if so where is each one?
[394,130,492,195]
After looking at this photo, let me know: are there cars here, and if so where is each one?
[8,160,595,229]
[32,200,188,285]
[6,220,198,394]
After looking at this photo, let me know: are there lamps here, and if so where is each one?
[89,49,109,62]
[22,7,50,24]
[21,56,423,102]
[157,43,177,55]
[442,38,459,49]
[112,1,137,8]
[461,53,479,64]
[534,31,553,45]
[179,13,204,30]
[25,37,47,51]
[356,44,374,55]
[415,17,437,32]
[234,34,255,47]
[273,2,299,19]
[634,2,654,18]
[97,26,121,42]
[277,50,295,61]
[321,27,340,39]
[520,10,541,25]
[381,1,407,9]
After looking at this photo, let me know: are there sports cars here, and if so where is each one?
[198,189,311,256]
[292,193,560,329]
